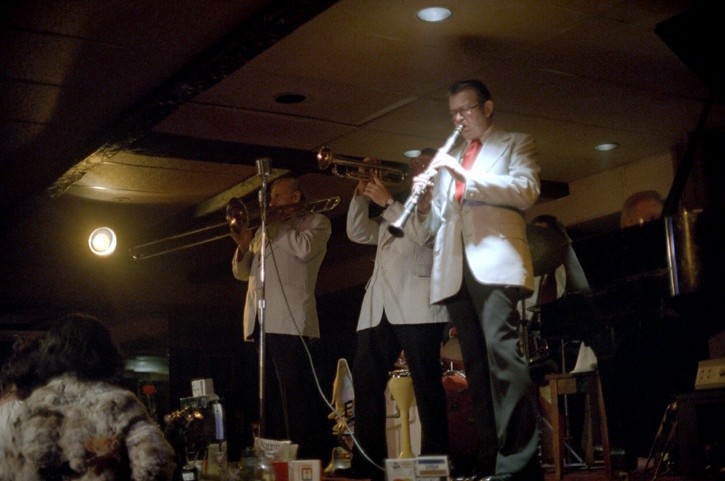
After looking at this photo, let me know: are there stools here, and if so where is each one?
[545,371,612,481]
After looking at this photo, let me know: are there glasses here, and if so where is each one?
[447,103,479,122]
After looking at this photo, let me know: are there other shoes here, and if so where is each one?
[458,475,545,481]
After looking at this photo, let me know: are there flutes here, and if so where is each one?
[387,124,464,239]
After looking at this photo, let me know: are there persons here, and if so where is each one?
[405,80,543,481]
[335,146,453,479]
[0,316,179,481]
[232,175,332,471]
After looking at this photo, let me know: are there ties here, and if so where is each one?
[456,139,483,205]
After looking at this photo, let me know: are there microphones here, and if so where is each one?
[256,158,273,207]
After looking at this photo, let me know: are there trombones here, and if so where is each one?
[128,195,341,262]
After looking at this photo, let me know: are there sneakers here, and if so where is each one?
[335,467,386,480]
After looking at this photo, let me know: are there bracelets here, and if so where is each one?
[385,196,396,209]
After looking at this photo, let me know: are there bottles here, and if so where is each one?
[258,457,276,481]
[182,466,195,481]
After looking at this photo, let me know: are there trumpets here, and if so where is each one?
[317,145,412,188]
